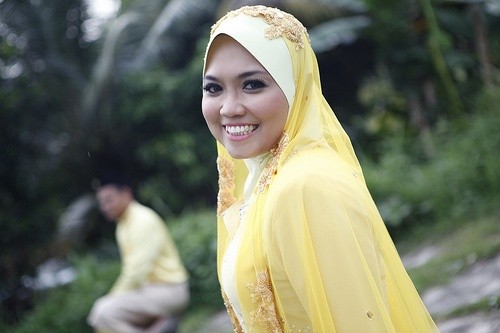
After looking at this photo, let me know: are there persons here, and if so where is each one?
[87,172,191,333]
[201,5,441,333]
